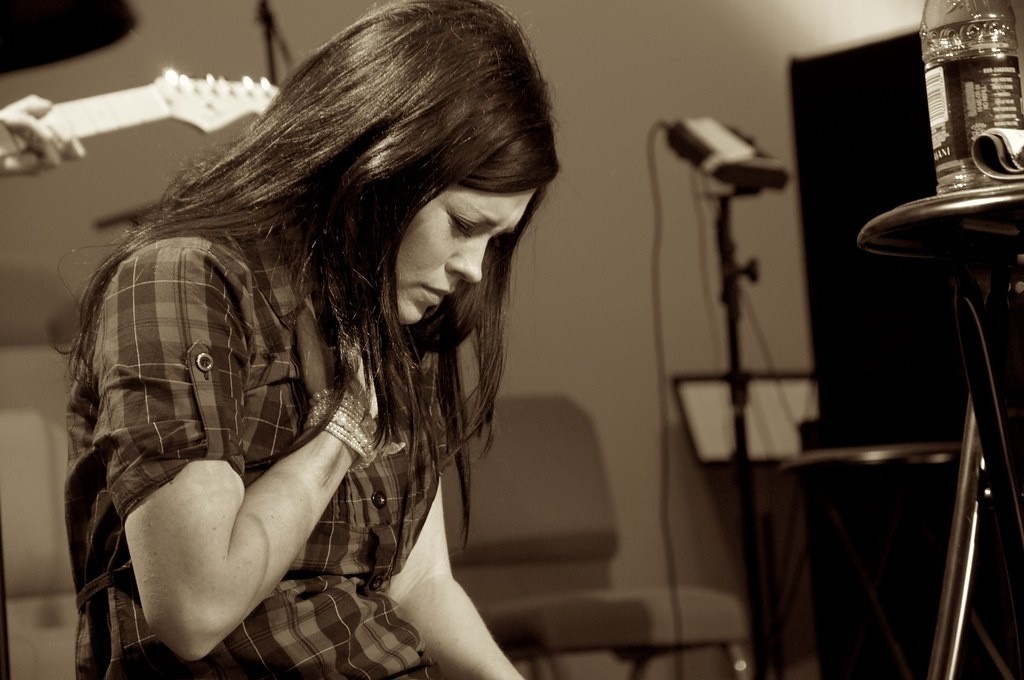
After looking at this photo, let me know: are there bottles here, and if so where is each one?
[919,0,1024,196]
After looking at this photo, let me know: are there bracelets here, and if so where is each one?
[304,387,382,472]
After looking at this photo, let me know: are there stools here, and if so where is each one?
[775,440,1012,680]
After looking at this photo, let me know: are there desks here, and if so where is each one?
[854,183,1024,680]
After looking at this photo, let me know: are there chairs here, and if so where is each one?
[443,394,745,680]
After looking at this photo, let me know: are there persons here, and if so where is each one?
[62,0,561,680]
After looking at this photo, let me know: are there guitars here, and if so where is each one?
[1,68,280,159]
